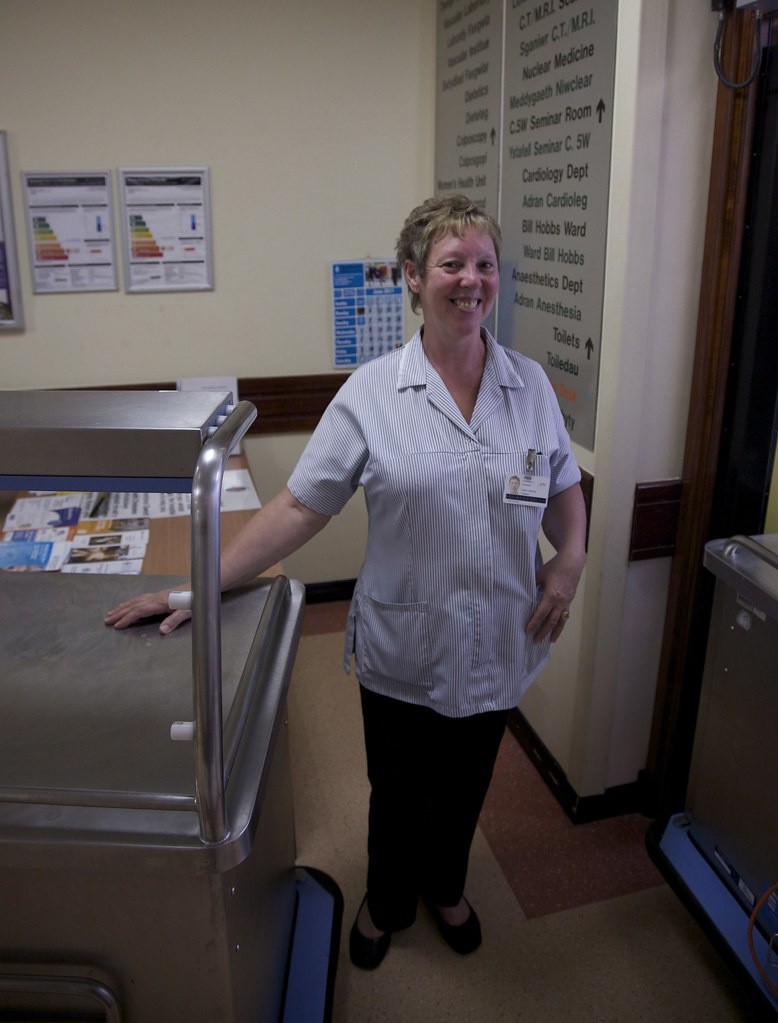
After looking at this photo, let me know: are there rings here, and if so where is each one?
[560,612,569,619]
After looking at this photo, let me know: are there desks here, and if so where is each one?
[0,446,283,579]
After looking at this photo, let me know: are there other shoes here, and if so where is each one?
[422,893,482,954]
[349,890,392,972]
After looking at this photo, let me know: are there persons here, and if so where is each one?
[508,476,520,495]
[104,195,587,972]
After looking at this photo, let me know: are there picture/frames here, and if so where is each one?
[0,128,27,332]
